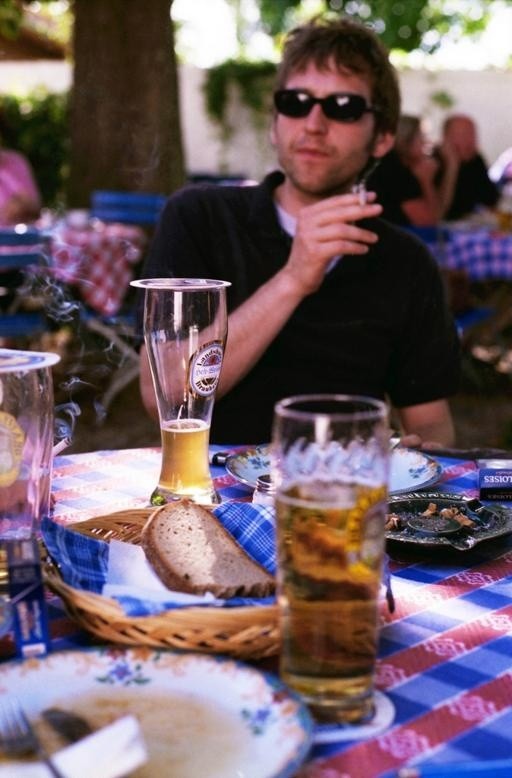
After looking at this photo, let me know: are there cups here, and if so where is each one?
[133,276,234,508]
[272,395,393,726]
[0,347,62,587]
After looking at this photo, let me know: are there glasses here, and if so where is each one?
[273,86,378,123]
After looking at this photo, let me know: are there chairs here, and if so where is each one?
[90,193,168,424]
[0,226,56,354]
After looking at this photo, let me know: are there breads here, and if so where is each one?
[142,498,275,598]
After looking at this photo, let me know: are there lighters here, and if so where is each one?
[205,451,234,466]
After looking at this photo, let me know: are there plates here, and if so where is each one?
[0,648,312,778]
[224,438,444,497]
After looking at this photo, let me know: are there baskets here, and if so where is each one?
[41,502,380,656]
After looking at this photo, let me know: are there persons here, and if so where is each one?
[357,113,444,242]
[411,113,507,222]
[133,16,472,454]
[1,479,58,517]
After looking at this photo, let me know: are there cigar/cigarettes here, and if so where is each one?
[49,435,73,457]
[357,179,368,206]
